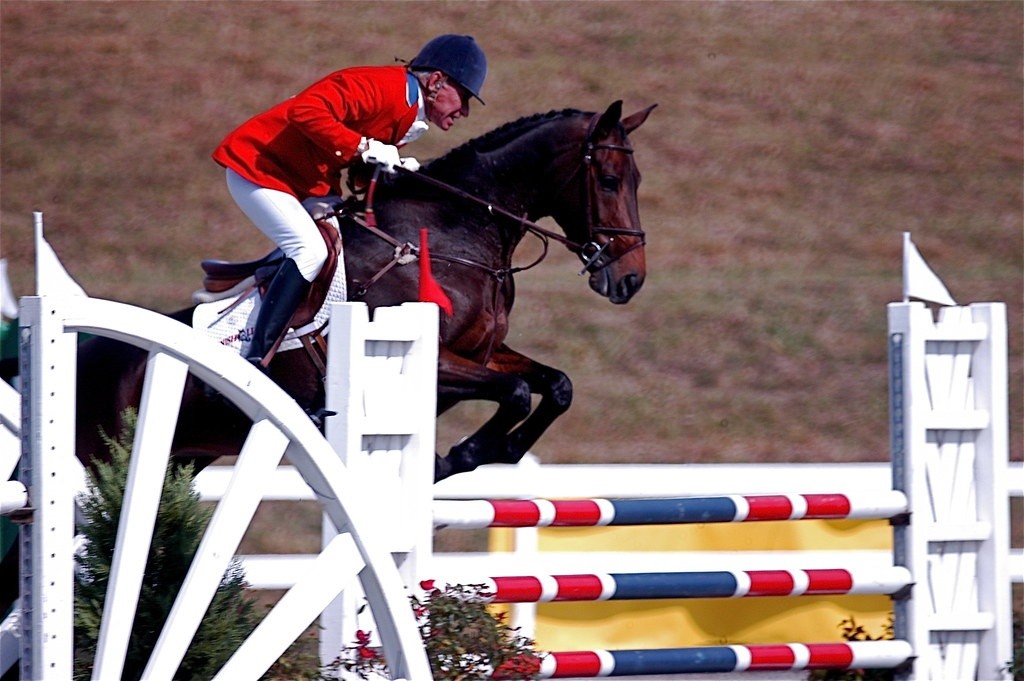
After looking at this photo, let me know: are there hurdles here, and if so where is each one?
[72,456,1024,681]
[320,300,1012,681]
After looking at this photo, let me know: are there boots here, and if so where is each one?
[244,258,310,403]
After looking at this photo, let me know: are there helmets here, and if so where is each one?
[410,34,487,106]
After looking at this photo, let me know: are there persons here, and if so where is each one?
[210,32,489,397]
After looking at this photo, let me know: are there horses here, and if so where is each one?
[76,98,660,487]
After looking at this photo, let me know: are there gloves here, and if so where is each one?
[361,138,401,173]
[400,158,421,172]
